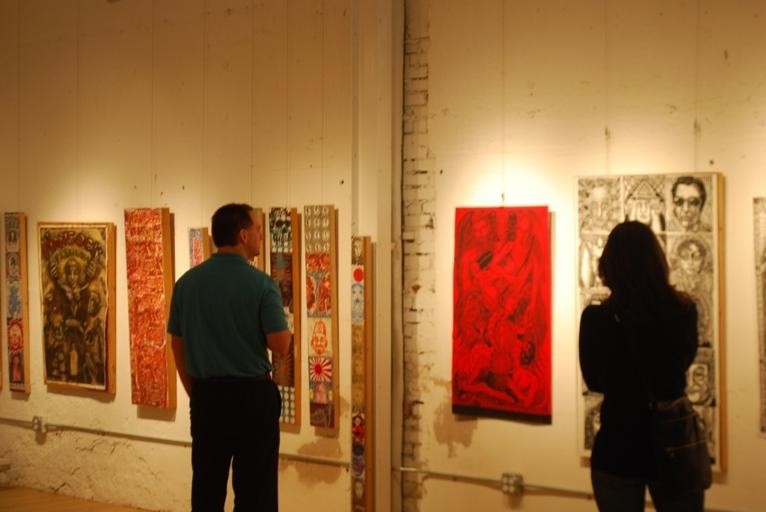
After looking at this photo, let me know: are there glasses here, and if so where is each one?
[674,197,700,207]
[680,251,702,261]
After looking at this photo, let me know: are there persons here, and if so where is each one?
[44,258,103,386]
[167,203,291,512]
[580,221,711,512]
[672,178,707,232]
[670,234,710,294]
[309,321,330,357]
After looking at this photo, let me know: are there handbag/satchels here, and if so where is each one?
[650,395,711,492]
